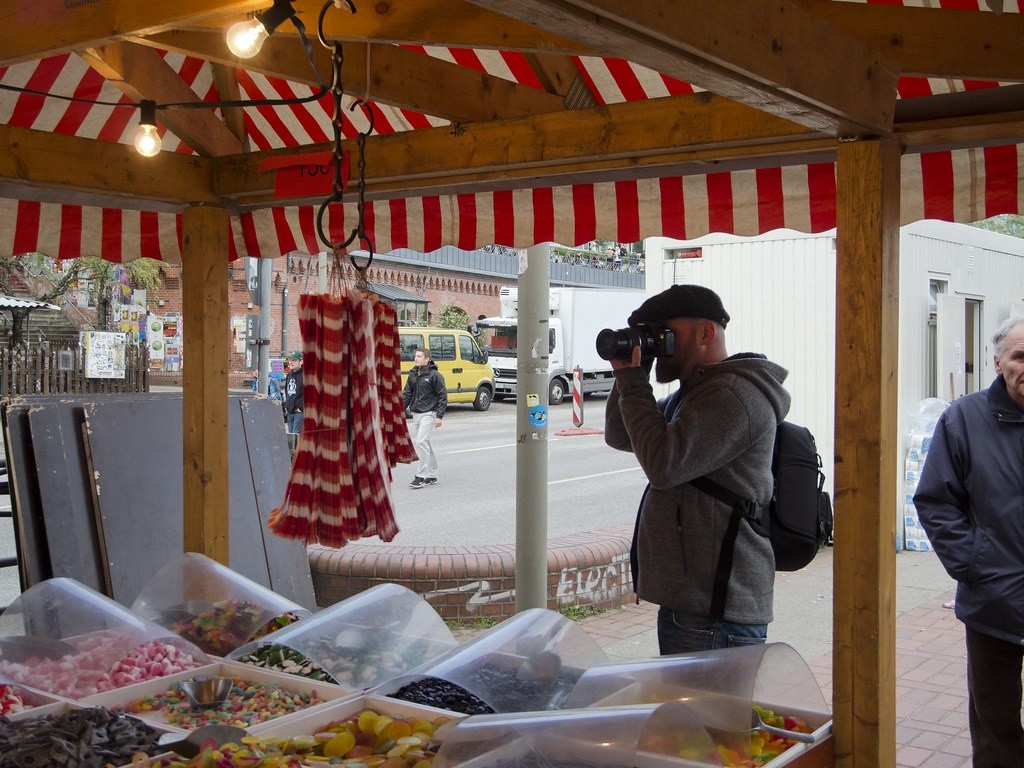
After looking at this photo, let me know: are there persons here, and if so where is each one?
[914,317,1024,768]
[402,349,447,488]
[252,370,283,403]
[285,351,303,462]
[606,284,791,699]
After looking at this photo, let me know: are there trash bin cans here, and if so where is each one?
[253,376,284,401]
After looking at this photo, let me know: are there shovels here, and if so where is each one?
[335,620,401,650]
[181,674,233,708]
[158,723,254,758]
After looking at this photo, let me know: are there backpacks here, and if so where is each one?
[700,366,832,624]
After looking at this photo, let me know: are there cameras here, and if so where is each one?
[596,321,675,361]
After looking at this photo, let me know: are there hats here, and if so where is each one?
[626,283,729,331]
[285,351,303,361]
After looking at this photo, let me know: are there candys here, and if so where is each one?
[0,596,814,768]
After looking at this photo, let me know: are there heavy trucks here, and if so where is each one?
[476,285,647,405]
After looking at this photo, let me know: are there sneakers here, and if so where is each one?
[409,475,425,488]
[422,478,438,486]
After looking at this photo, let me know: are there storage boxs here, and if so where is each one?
[0,598,834,768]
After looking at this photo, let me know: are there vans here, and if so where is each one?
[397,325,497,412]
